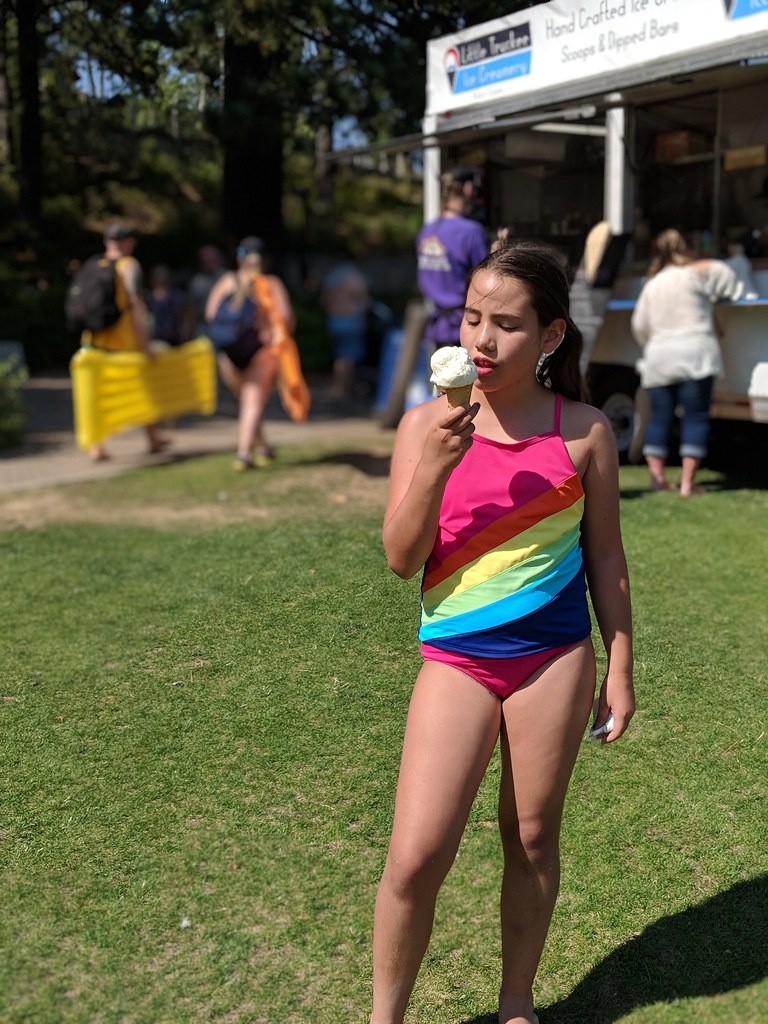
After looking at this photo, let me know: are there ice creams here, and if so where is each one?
[430,346,478,414]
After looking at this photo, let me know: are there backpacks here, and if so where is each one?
[64,254,132,331]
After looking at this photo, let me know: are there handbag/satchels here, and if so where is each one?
[208,272,258,349]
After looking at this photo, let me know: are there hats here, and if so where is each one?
[104,224,138,239]
[239,237,263,253]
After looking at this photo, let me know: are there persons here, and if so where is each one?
[371,243,639,1024]
[66,170,753,497]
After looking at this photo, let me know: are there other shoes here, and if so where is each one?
[257,445,276,461]
[232,456,255,472]
